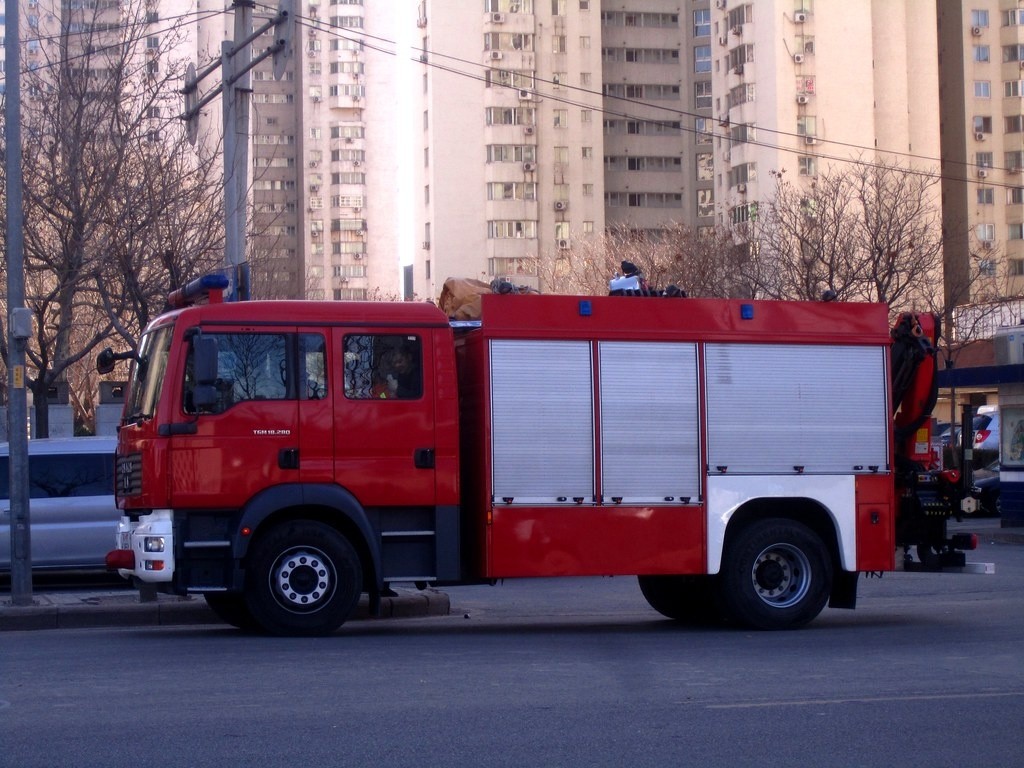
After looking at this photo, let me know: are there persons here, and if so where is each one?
[342,344,422,399]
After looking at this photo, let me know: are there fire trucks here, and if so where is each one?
[96,261,979,640]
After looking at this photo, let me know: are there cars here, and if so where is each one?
[940,424,961,445]
[973,459,1001,516]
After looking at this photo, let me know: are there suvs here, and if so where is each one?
[957,411,1000,451]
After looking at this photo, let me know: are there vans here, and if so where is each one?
[0,436,120,575]
